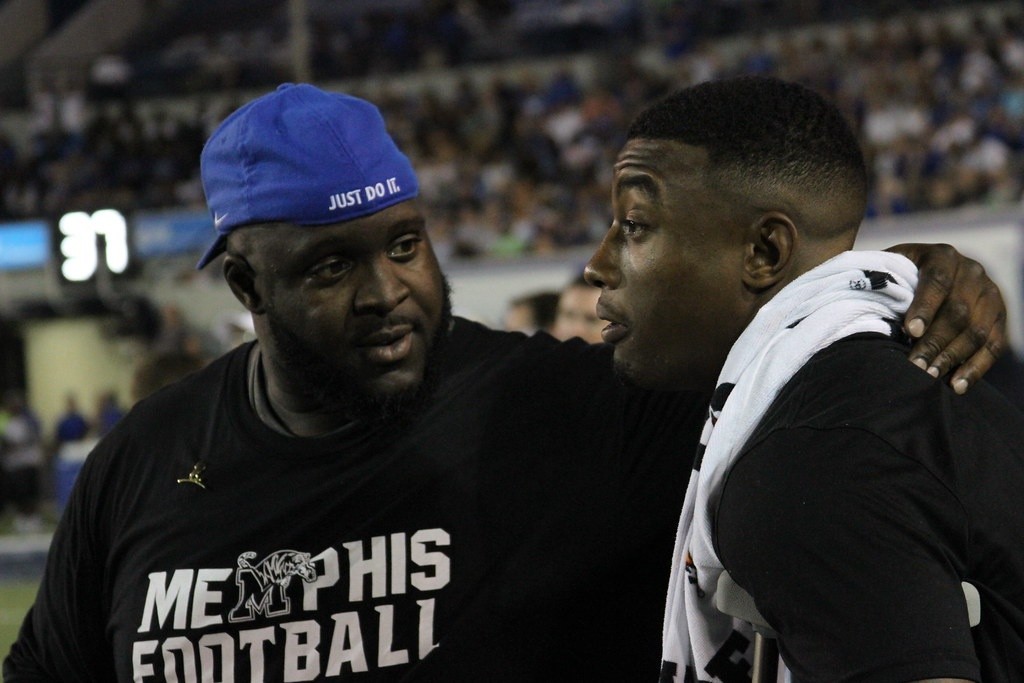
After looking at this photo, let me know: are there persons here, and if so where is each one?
[0,0,1024,525]
[582,73,1024,683]
[2,81,1007,682]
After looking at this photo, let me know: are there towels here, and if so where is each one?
[658,247,921,683]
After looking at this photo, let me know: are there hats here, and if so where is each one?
[195,83,418,271]
